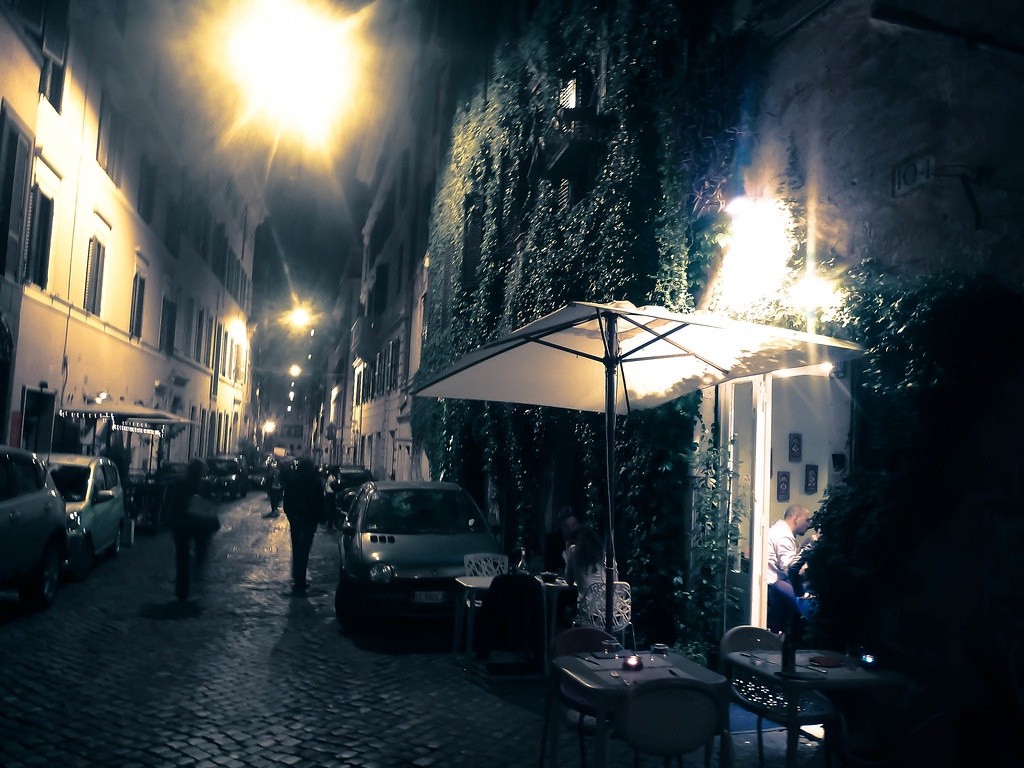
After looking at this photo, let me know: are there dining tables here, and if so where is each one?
[534,575,578,650]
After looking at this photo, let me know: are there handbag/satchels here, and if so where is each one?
[174,495,220,534]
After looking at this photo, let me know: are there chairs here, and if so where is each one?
[463,552,509,610]
[720,625,833,768]
[835,709,993,768]
[608,677,721,768]
[538,627,624,768]
[475,573,548,676]
[572,581,637,650]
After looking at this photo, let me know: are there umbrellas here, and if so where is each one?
[409,296,866,635]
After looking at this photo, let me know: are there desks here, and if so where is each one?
[454,575,494,669]
[723,650,907,768]
[548,651,734,768]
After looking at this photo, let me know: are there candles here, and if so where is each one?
[622,654,643,672]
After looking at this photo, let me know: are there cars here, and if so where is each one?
[29,452,126,567]
[335,480,507,624]
[319,463,375,528]
[146,454,270,501]
[0,444,68,614]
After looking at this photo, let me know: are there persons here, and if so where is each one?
[768,504,823,627]
[264,453,342,592]
[167,457,219,606]
[548,512,625,641]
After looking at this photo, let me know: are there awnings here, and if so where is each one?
[67,400,198,429]
